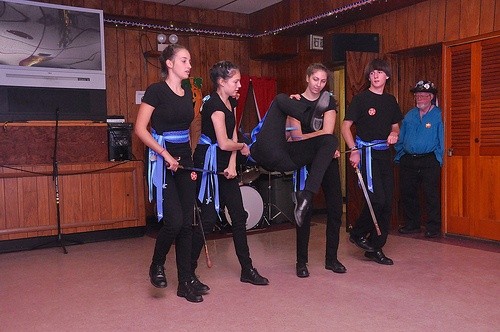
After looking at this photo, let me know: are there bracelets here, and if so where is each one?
[240,143,245,150]
[159,148,165,154]
[349,145,358,150]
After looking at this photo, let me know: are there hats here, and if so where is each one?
[410,80,436,94]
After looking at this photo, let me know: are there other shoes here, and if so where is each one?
[398,225,420,233]
[424,230,440,238]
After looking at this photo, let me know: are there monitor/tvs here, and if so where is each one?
[0,0,107,89]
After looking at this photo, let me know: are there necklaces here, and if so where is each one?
[419,104,432,124]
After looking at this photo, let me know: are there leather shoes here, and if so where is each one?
[240,267,270,285]
[296,261,309,277]
[304,91,330,132]
[349,232,374,252]
[190,273,211,295]
[291,191,309,228]
[149,261,167,289]
[177,281,203,302]
[324,258,347,273]
[364,248,394,264]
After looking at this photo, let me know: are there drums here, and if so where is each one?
[219,185,264,232]
[236,162,262,184]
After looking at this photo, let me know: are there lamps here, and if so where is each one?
[157,33,178,51]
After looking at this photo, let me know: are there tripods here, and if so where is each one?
[262,173,295,226]
[30,108,84,254]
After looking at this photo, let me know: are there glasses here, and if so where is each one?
[415,94,431,99]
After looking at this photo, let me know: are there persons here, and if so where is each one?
[341,58,404,265]
[247,91,339,228]
[193,60,270,293]
[290,64,346,278]
[392,80,445,237]
[134,43,204,303]
